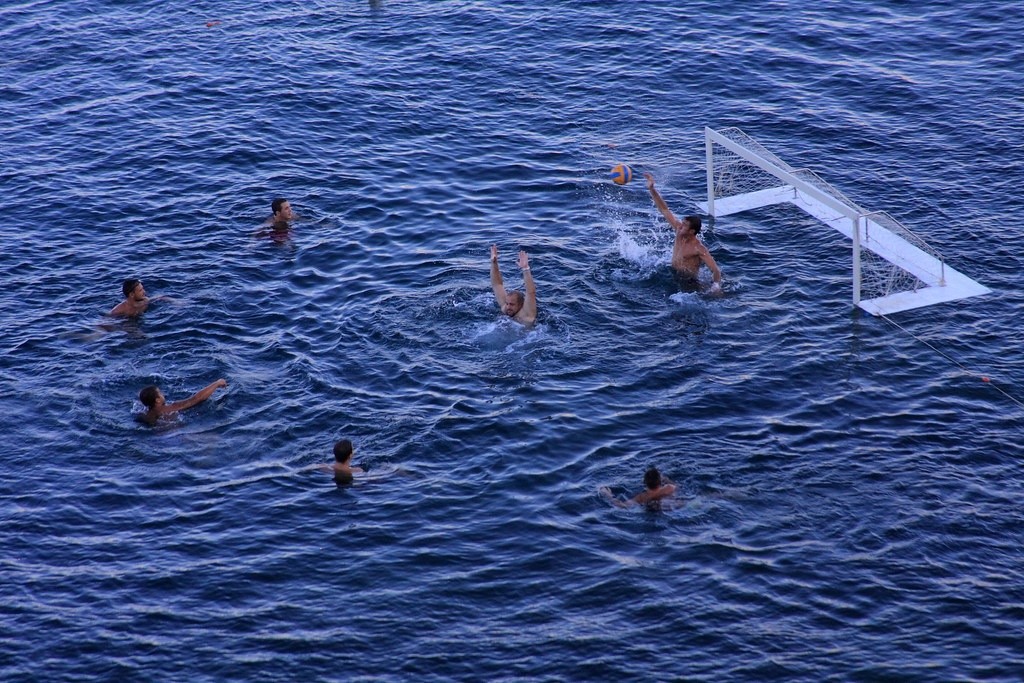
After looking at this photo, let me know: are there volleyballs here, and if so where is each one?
[609,163,632,185]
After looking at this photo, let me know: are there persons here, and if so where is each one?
[333,440,363,473]
[643,469,675,499]
[490,244,537,323]
[271,198,292,220]
[643,172,721,283]
[139,378,227,415]
[111,278,149,315]
